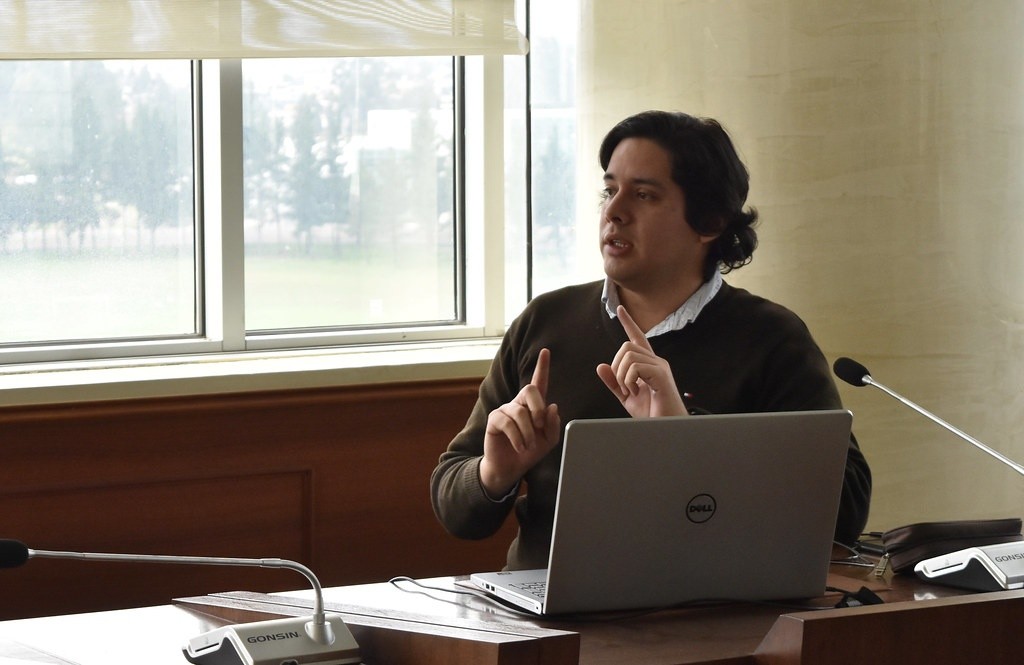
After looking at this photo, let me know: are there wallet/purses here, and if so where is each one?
[875,518,1024,576]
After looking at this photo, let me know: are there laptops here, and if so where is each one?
[469,410,852,618]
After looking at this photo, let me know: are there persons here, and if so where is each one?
[429,110,873,571]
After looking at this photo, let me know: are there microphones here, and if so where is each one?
[1,537,363,663]
[833,356,1023,476]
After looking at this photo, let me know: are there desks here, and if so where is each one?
[1,538,1024,665]
